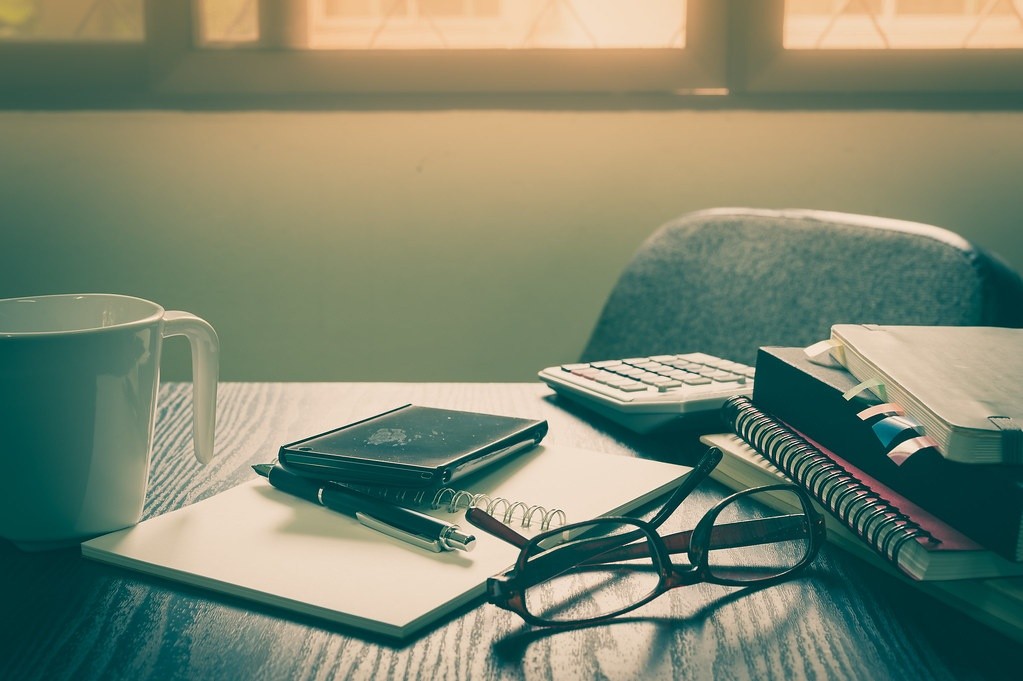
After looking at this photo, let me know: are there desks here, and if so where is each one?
[0,382,1023,681]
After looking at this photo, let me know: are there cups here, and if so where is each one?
[0,291,220,552]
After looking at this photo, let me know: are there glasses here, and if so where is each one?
[464,446,825,628]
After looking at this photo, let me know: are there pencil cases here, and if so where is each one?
[250,463,478,554]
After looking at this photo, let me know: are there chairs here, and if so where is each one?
[577,209,1023,368]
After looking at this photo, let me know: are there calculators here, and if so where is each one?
[538,352,756,437]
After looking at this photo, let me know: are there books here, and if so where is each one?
[82,443,696,638]
[700,325,1023,641]
